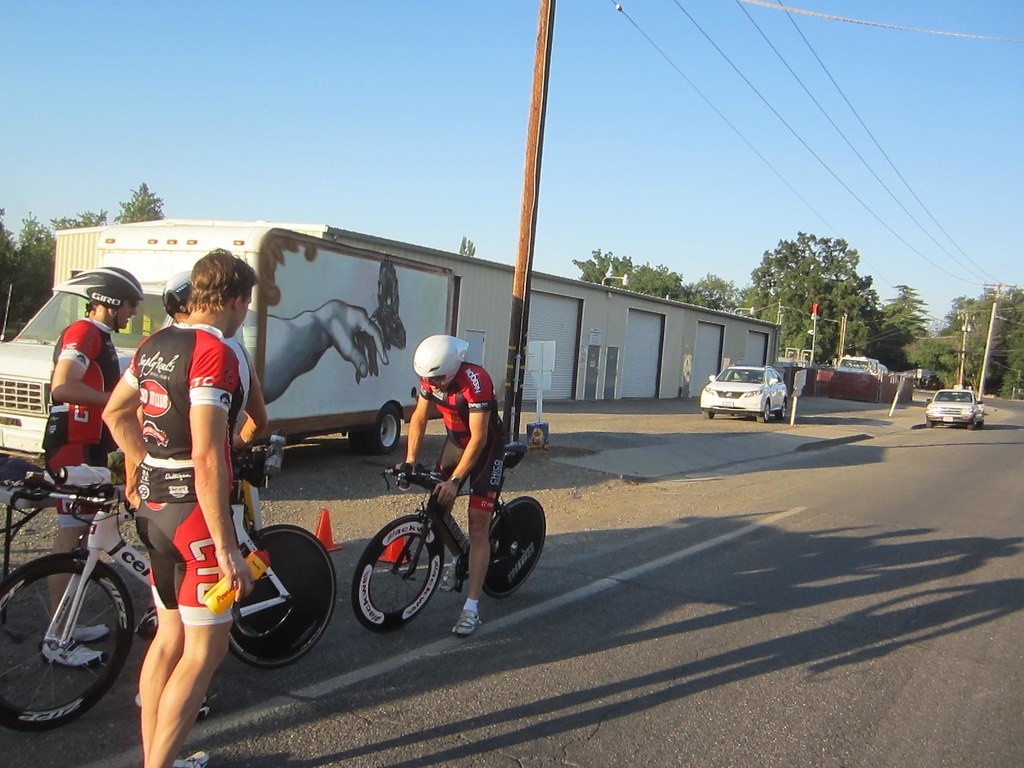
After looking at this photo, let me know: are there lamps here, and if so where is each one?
[602,273,631,288]
[734,306,755,317]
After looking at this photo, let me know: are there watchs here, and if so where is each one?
[452,477,461,484]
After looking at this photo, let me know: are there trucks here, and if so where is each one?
[0,219,457,473]
[835,357,889,381]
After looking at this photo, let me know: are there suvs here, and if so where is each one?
[700,361,787,423]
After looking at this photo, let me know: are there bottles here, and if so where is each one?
[61,466,113,486]
[202,549,271,615]
[263,430,286,475]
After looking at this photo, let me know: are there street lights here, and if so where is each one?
[807,330,815,366]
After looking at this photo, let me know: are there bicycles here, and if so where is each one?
[351,443,546,633]
[0,452,337,733]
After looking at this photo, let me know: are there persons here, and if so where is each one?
[396,334,504,637]
[101,250,258,768]
[126,272,268,640]
[41,267,143,667]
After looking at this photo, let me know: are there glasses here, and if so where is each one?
[120,297,140,307]
[435,375,445,381]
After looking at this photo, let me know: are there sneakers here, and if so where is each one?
[43,640,108,669]
[173,751,210,768]
[72,621,109,643]
[134,607,158,635]
[193,690,218,721]
[451,609,480,634]
[439,564,457,592]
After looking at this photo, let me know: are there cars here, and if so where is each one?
[912,376,940,389]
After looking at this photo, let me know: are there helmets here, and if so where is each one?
[162,270,192,316]
[51,267,144,308]
[414,334,460,386]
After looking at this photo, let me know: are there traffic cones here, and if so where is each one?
[376,534,409,563]
[312,508,341,554]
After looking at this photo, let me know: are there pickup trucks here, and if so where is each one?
[924,389,985,429]
